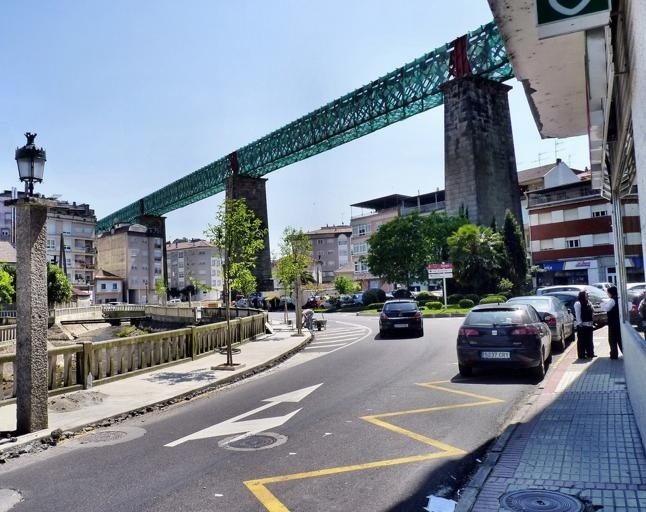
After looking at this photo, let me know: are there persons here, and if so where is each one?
[573,291,597,360]
[599,287,623,359]
[301,309,314,330]
[637,292,646,340]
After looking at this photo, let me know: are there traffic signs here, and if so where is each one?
[428,263,452,268]
[428,274,453,279]
[428,268,453,273]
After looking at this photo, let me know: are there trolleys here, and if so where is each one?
[313,311,327,330]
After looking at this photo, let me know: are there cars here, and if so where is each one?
[504,295,576,353]
[167,299,181,303]
[627,282,646,340]
[535,282,617,328]
[230,291,363,310]
[106,302,122,306]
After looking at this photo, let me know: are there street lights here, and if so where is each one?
[14,132,48,435]
[86,283,90,294]
[316,255,323,287]
[144,280,148,304]
[292,258,302,330]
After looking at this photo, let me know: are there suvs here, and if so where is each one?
[377,297,425,337]
[456,299,553,380]
